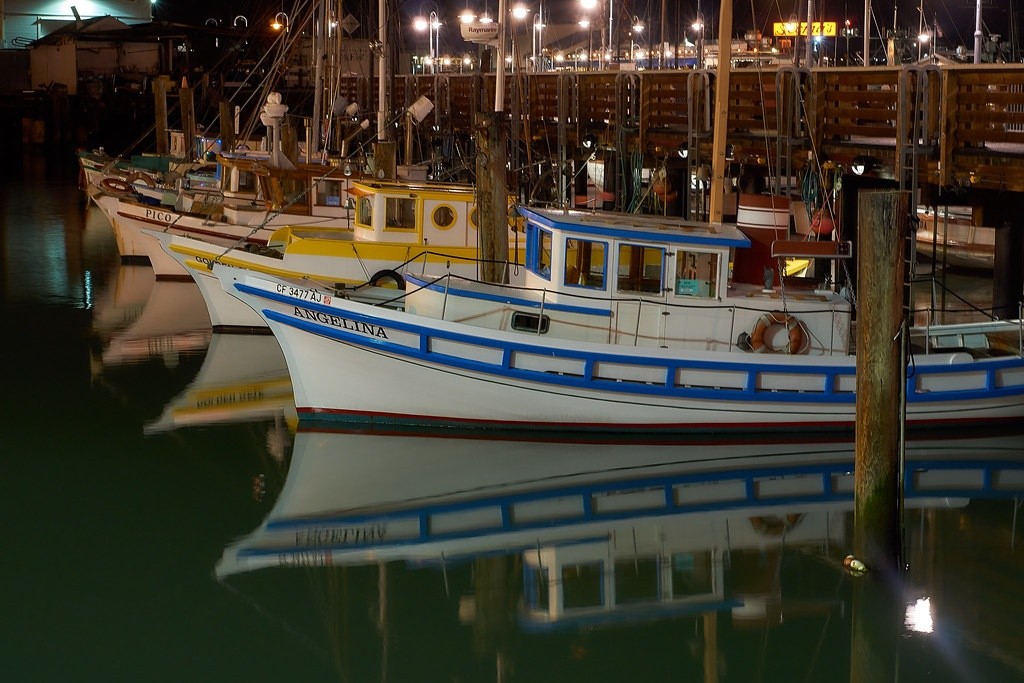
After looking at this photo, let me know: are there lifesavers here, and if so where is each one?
[127,171,154,188]
[750,512,803,539]
[751,312,803,355]
[101,178,130,192]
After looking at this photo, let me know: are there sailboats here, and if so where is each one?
[212,415,1024,682]
[68,0,986,336]
[212,0,1024,435]
[93,260,300,462]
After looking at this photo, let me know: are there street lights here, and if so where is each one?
[271,12,289,35]
[629,15,644,63]
[429,10,441,75]
[531,13,545,73]
[920,24,931,62]
[786,13,799,66]
